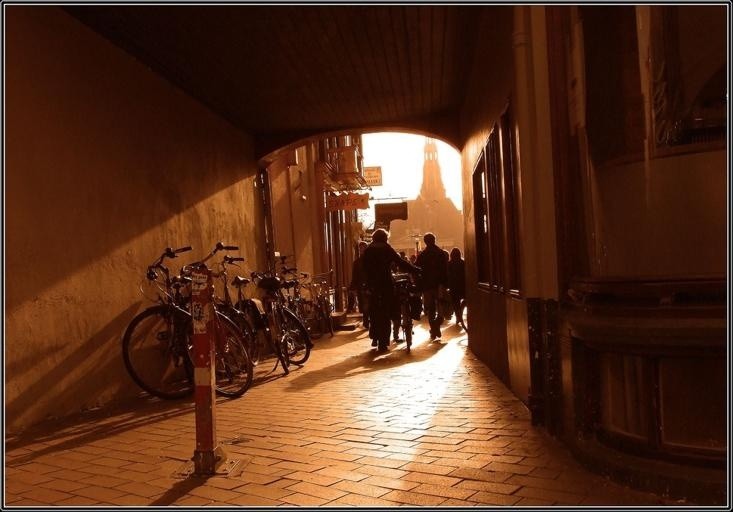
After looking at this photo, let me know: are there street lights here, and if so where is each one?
[411,232,423,258]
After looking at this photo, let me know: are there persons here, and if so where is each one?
[349,228,464,352]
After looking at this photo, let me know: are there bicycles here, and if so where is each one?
[165,242,334,398]
[122,246,255,400]
[382,269,424,353]
[458,298,467,333]
